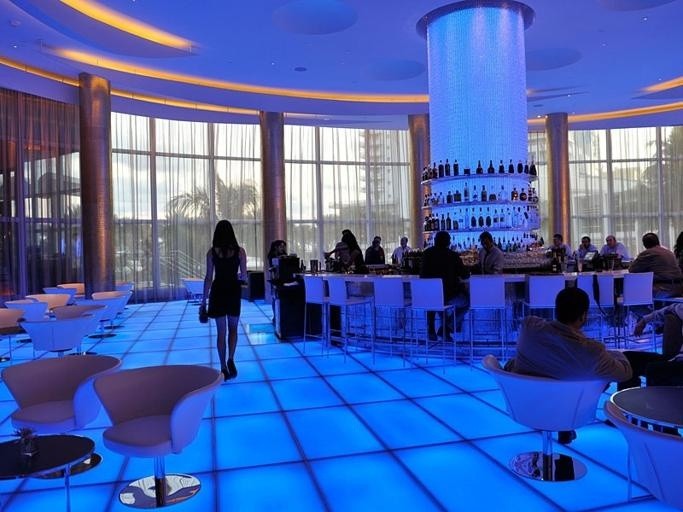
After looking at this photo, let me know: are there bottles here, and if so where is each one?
[419,158,543,256]
[548,247,567,272]
[295,258,324,274]
[401,247,425,258]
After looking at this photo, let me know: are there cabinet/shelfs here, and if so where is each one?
[420,173,543,255]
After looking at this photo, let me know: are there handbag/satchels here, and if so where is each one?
[199,303,208,322]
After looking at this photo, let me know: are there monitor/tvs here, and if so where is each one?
[584,250,598,261]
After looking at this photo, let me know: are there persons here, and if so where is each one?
[604,301,682,428]
[572,236,598,262]
[474,231,504,275]
[391,237,412,267]
[503,287,633,445]
[419,231,470,342]
[541,233,572,261]
[599,235,629,261]
[268,240,288,322]
[199,220,248,380]
[629,233,682,299]
[364,236,385,265]
[323,228,369,275]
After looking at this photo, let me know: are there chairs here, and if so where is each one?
[92,365,225,509]
[1,282,133,359]
[605,387,681,511]
[2,355,123,480]
[481,355,611,483]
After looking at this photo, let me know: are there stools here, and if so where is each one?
[372,278,418,368]
[576,274,618,350]
[411,278,457,374]
[470,276,514,364]
[302,275,332,356]
[522,275,565,322]
[325,277,371,360]
[615,271,654,352]
[652,298,683,353]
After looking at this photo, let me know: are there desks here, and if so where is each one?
[183,278,210,306]
[0,426,97,512]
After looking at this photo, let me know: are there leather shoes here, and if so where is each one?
[557,431,575,444]
[227,359,236,379]
[436,328,453,342]
[220,367,229,381]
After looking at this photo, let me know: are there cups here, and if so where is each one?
[502,247,551,270]
